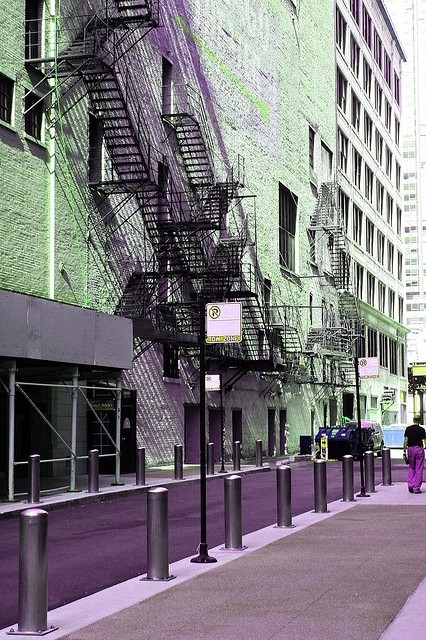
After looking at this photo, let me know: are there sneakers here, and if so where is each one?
[415,491,422,493]
[409,487,413,493]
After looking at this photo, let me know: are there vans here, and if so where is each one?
[345,421,386,457]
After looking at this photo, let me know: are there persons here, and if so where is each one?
[403,415,426,494]
